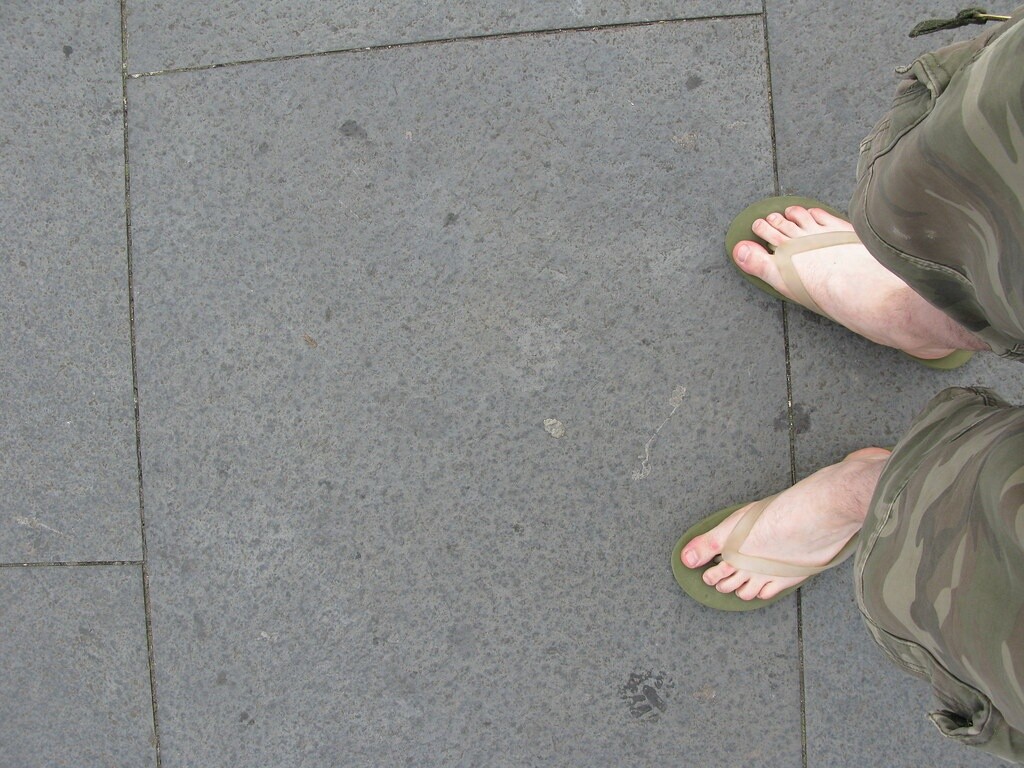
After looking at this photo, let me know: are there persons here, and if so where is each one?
[670,7,1024,768]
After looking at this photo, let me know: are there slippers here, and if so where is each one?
[726,195,976,370]
[670,446,896,613]
